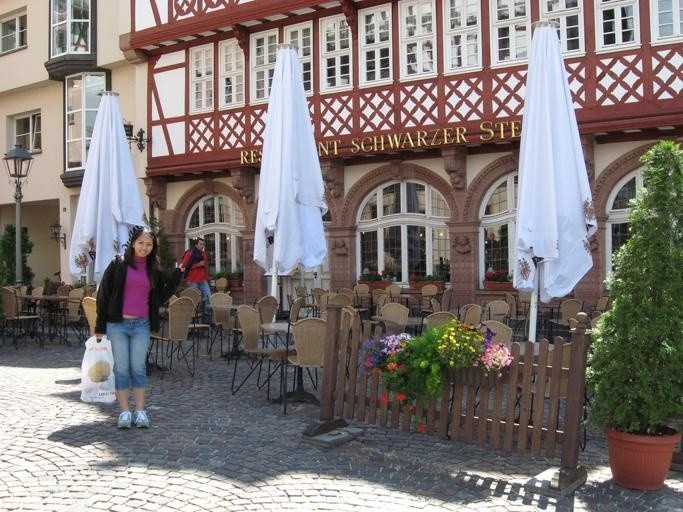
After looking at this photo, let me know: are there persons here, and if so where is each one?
[181,237,212,338]
[95,226,183,428]
[487,233,497,249]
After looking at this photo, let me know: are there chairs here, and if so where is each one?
[210,282,615,415]
[3,279,203,382]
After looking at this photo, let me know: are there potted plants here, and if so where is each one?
[582,141,682,493]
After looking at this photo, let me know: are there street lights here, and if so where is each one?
[2,142,35,290]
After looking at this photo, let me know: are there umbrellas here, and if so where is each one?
[512,19,599,344]
[253,42,328,324]
[69,90,152,291]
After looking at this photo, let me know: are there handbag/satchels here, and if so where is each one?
[79,335,117,403]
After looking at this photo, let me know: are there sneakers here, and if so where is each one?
[117,410,132,428]
[132,410,149,428]
